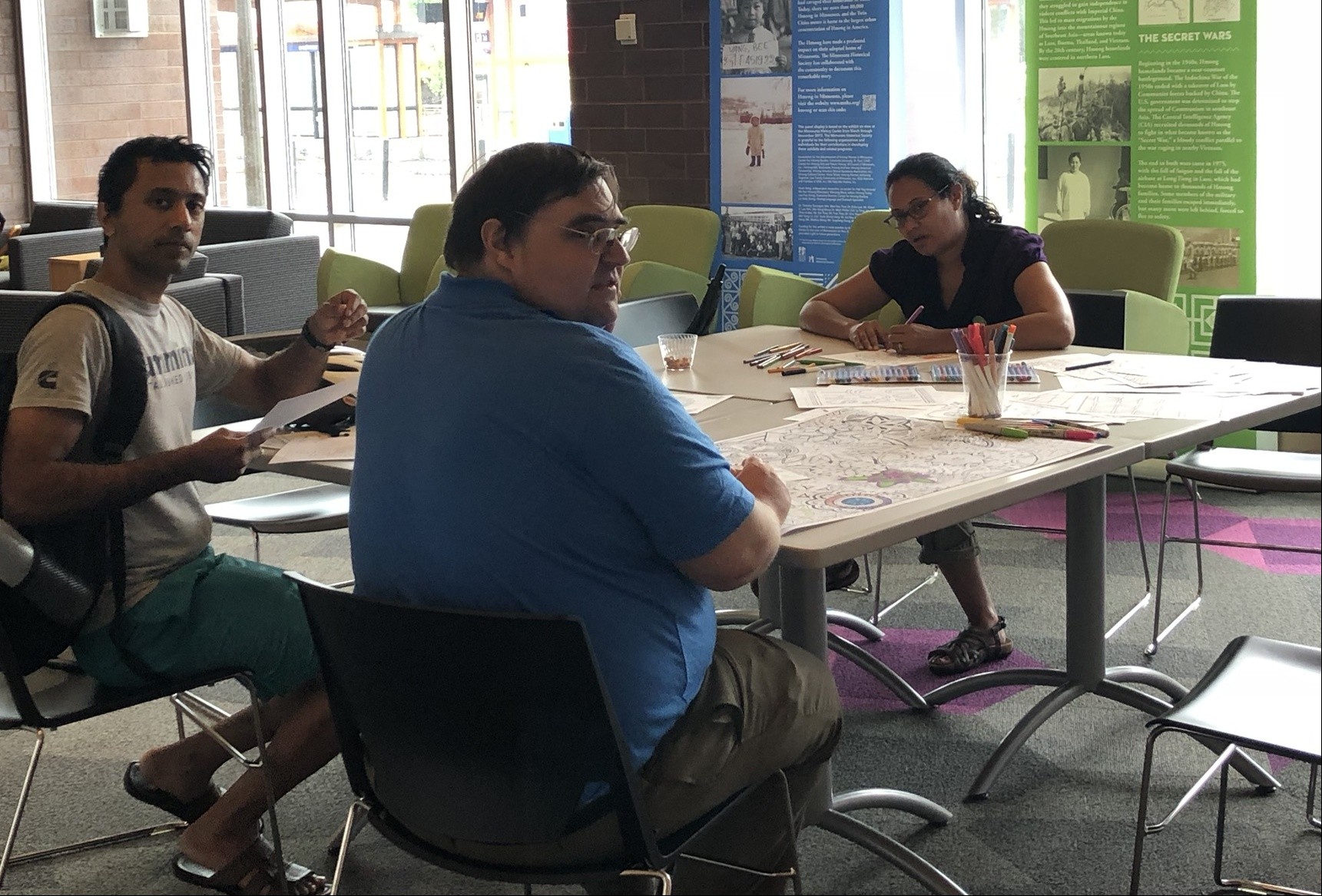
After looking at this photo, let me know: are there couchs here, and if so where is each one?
[1,203,319,424]
[322,203,1192,639]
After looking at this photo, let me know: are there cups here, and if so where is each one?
[657,334,698,371]
[956,349,1013,420]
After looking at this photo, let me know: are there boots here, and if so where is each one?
[757,155,761,167]
[750,156,755,167]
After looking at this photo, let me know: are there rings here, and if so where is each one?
[897,343,903,351]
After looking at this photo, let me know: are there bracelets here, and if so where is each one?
[301,319,337,352]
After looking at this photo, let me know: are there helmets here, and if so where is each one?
[301,345,364,431]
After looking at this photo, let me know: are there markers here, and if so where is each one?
[834,366,918,382]
[957,413,1109,440]
[931,361,1032,381]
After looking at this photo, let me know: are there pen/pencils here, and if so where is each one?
[780,344,809,358]
[768,367,804,373]
[744,353,772,363]
[782,360,796,368]
[950,322,1016,419]
[1065,360,1113,371]
[782,363,845,376]
[757,356,777,368]
[753,345,778,357]
[750,356,773,366]
[885,305,923,354]
[797,359,842,364]
[794,348,815,359]
[806,348,823,356]
[761,355,781,369]
[770,342,800,353]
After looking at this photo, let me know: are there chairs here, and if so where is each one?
[283,568,802,896]
[205,483,356,588]
[1143,295,1322,658]
[0,518,288,896]
[868,466,1151,627]
[1132,636,1321,896]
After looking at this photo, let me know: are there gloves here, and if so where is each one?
[762,149,765,158]
[746,145,750,155]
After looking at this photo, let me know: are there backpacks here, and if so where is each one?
[0,290,149,677]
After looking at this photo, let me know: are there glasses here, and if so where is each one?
[515,211,641,255]
[883,184,950,229]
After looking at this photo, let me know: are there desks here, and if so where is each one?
[190,325,1322,896]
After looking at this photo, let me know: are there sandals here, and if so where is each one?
[927,616,1013,675]
[750,558,860,599]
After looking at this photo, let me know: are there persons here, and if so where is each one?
[720,0,788,75]
[1,134,371,895]
[1056,152,1091,220]
[1112,168,1130,219]
[1038,74,1131,142]
[721,210,794,259]
[744,115,765,166]
[750,152,1076,676]
[345,141,843,895]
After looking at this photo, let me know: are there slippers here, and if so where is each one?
[171,832,333,896]
[122,759,263,833]
